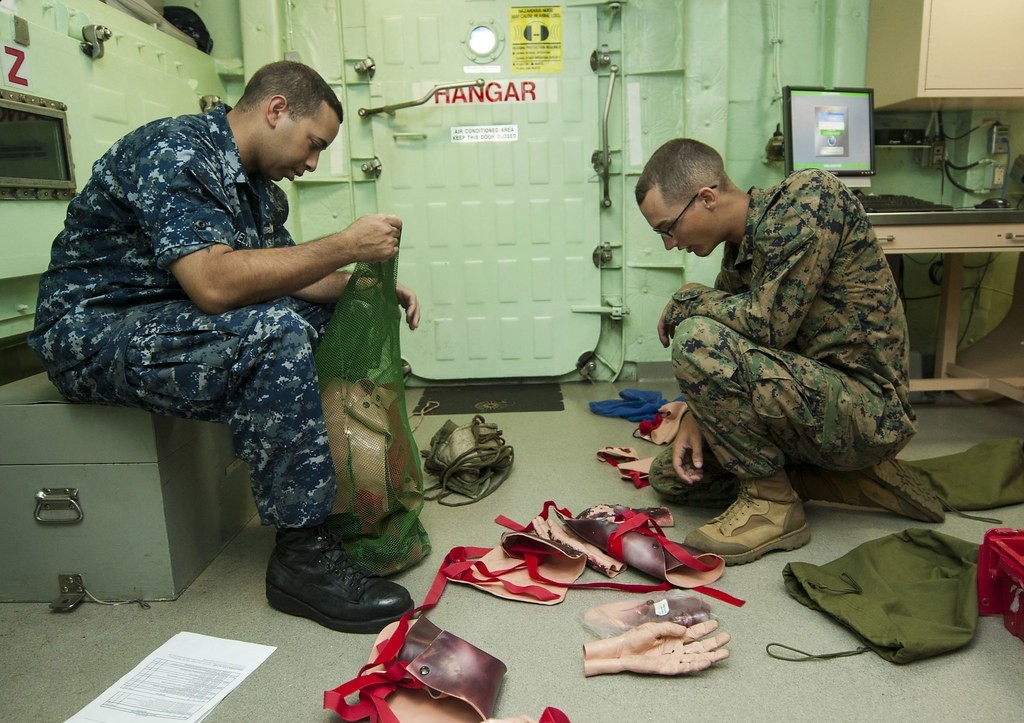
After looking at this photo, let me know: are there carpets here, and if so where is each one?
[414,383,565,415]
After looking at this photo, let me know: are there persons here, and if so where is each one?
[635,137,946,564]
[31,61,418,633]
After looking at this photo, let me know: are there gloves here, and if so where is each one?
[582,619,730,678]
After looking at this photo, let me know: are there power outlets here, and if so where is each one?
[984,164,1005,189]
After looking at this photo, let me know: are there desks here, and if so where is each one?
[872,221,1024,405]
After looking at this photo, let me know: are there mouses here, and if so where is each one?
[980,198,1006,208]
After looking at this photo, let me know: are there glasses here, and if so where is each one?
[651,185,718,239]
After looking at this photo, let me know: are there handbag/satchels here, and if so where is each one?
[765,528,983,666]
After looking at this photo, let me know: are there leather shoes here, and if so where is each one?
[264,520,416,634]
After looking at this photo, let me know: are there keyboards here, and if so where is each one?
[857,194,954,213]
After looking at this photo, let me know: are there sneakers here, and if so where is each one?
[683,468,811,567]
[824,456,947,524]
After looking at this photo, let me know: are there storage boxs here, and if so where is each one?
[0,368,256,604]
[977,528,1024,640]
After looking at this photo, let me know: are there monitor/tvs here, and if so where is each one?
[782,85,876,177]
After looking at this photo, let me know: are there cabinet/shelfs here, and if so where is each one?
[864,0,1024,115]
